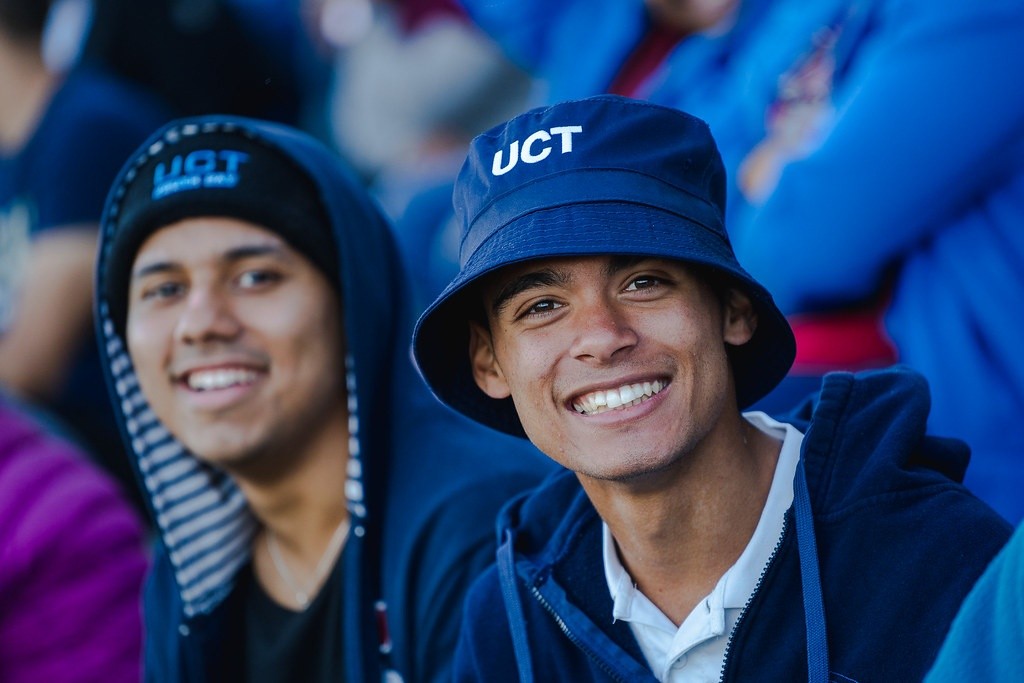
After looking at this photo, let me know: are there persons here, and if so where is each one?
[0,0,1024,683]
[413,94,1015,683]
[94,116,566,683]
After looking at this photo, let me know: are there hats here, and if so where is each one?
[106,132,338,343]
[409,97,798,439]
